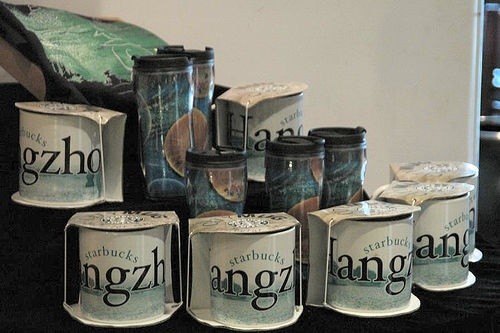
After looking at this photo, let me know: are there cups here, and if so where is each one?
[131,54,193,202]
[325,210,414,312]
[390,160,480,255]
[264,136,325,260]
[78,225,167,324]
[208,225,295,328]
[307,126,368,211]
[19,107,104,203]
[226,91,305,179]
[385,190,470,287]
[183,149,247,218]
[156,45,215,153]
